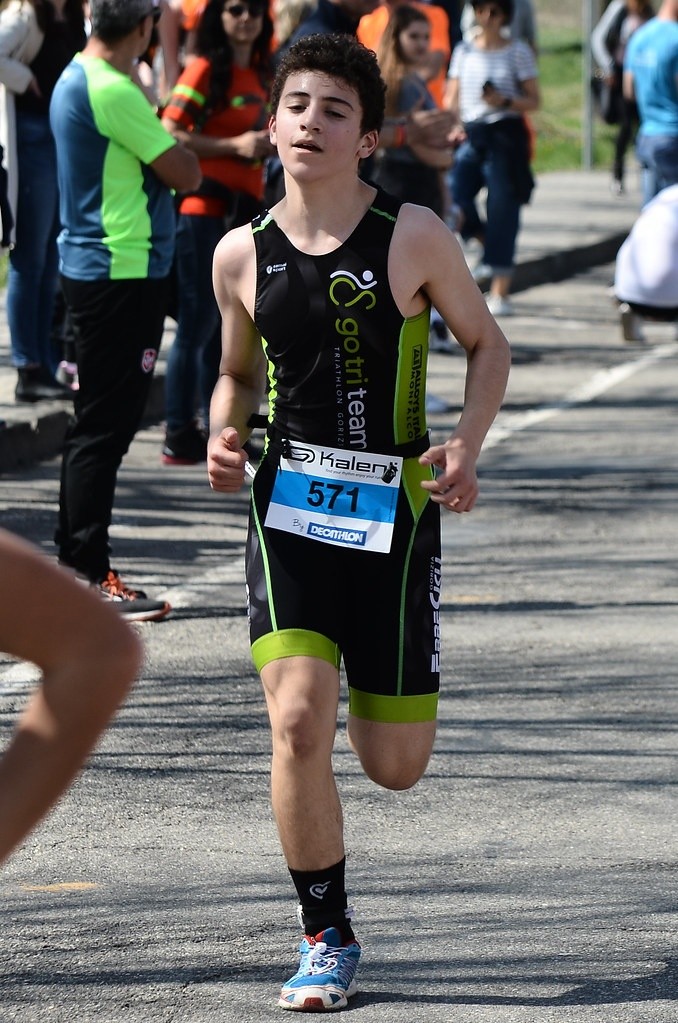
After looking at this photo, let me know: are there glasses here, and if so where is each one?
[222,0,263,19]
[139,7,162,27]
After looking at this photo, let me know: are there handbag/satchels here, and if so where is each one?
[597,61,626,126]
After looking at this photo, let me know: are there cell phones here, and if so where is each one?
[483,81,495,96]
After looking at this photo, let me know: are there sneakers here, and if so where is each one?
[277,924,361,1012]
[73,565,172,623]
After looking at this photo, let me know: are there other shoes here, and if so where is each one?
[620,306,646,344]
[57,362,81,388]
[14,368,76,400]
[161,440,207,466]
[487,294,511,317]
[239,439,267,462]
[429,322,452,351]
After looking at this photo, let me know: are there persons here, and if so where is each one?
[206,32,511,1010]
[0,529,143,864]
[588,0,678,341]
[0,0,542,623]
